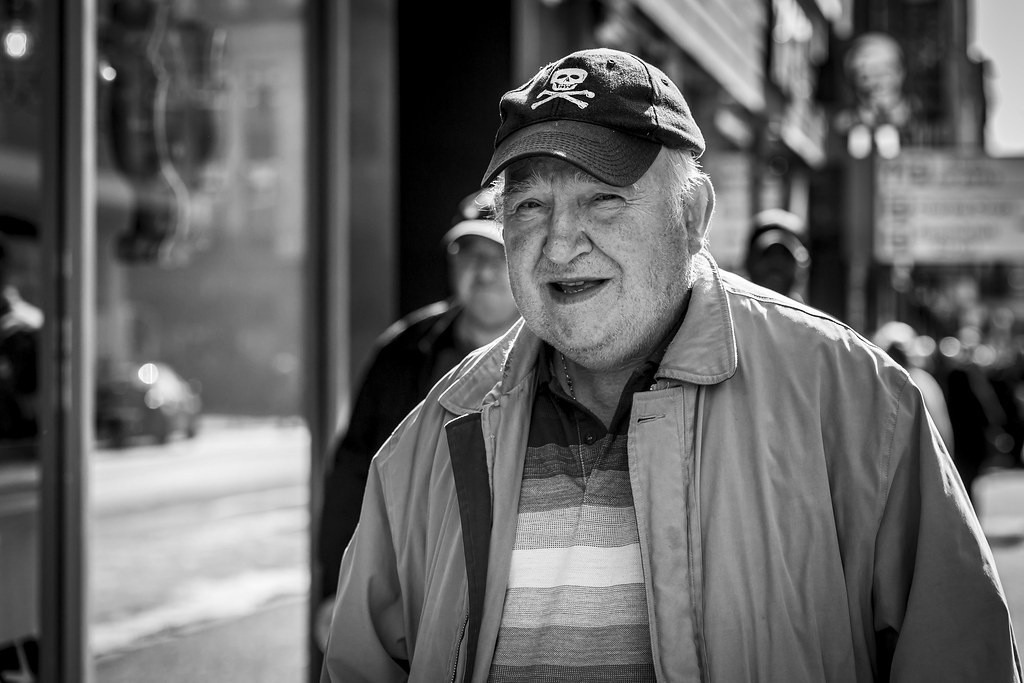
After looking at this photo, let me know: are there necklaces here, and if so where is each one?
[560,352,579,402]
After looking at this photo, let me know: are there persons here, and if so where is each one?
[314,188,521,654]
[321,47,1024,683]
[749,213,1024,507]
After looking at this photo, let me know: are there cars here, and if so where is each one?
[92,355,203,447]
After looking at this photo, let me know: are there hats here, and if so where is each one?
[445,191,506,249]
[480,47,706,190]
